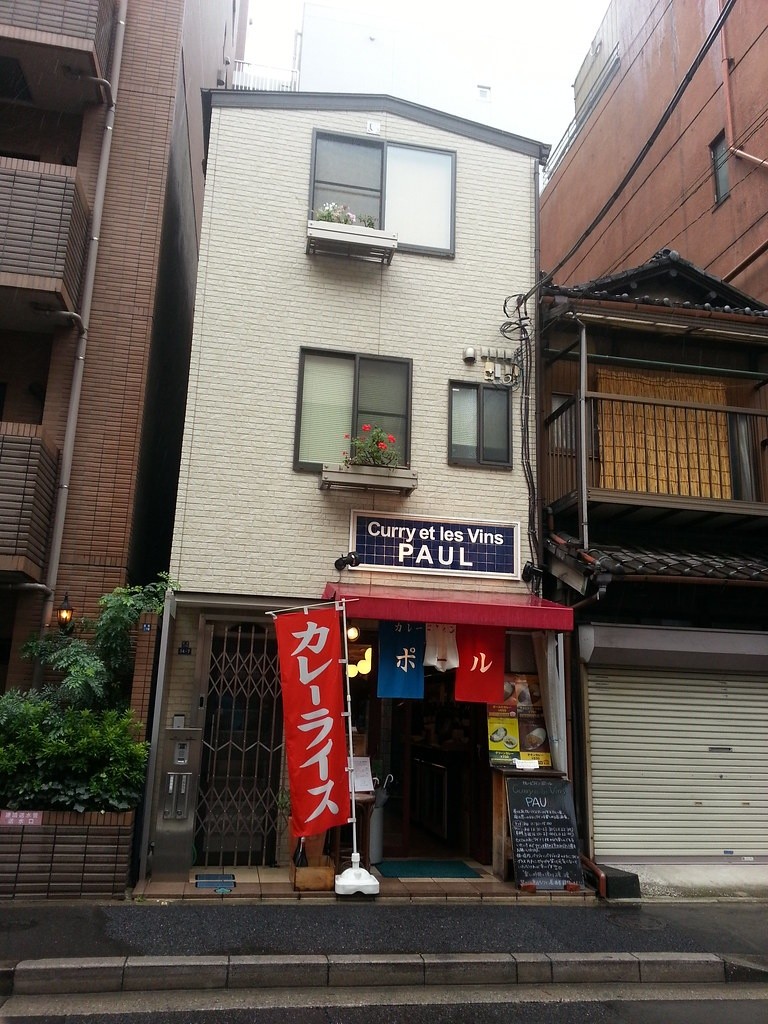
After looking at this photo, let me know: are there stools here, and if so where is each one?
[334,792,375,874]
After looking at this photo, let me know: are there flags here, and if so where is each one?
[377,620,425,698]
[275,608,351,836]
[455,624,504,703]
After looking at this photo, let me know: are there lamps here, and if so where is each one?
[57,592,75,636]
[334,552,360,571]
[522,562,548,583]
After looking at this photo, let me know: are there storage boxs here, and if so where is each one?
[369,808,383,864]
[289,854,334,892]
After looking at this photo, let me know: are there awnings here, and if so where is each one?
[324,582,574,630]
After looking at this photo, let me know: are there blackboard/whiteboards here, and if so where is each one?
[505,776,586,892]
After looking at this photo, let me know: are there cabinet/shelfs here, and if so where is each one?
[411,743,463,845]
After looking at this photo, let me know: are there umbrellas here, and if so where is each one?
[369,774,395,808]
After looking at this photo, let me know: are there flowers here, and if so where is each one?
[339,421,401,469]
[317,202,357,224]
